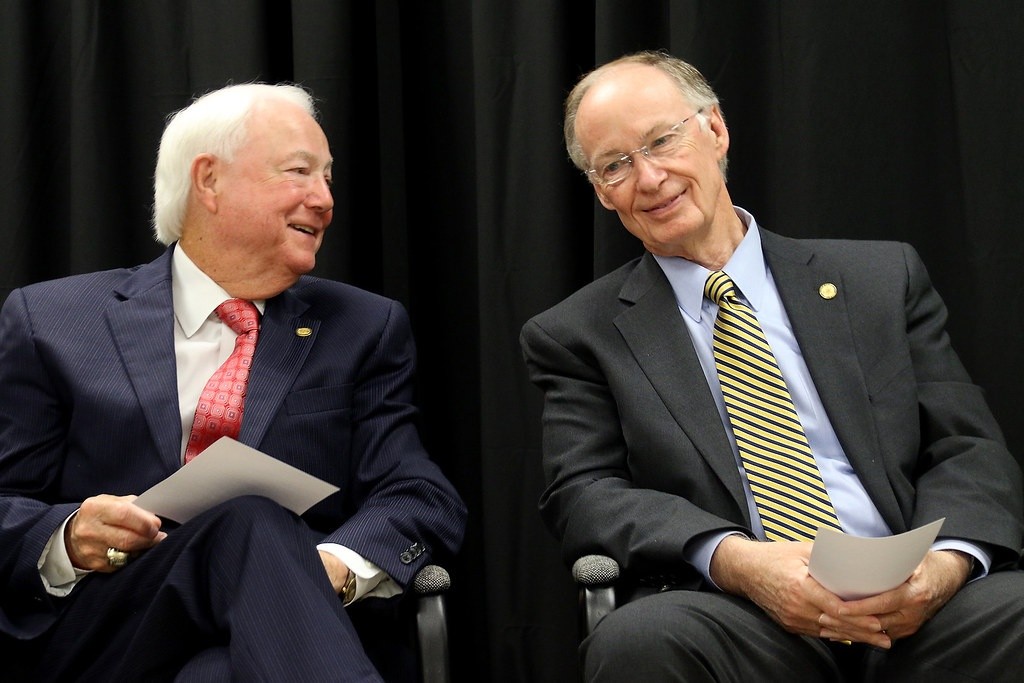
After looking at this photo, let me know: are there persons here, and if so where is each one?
[518,52,1024,683]
[0,82,470,683]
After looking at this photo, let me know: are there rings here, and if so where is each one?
[107,548,128,566]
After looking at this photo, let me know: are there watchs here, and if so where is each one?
[342,570,356,605]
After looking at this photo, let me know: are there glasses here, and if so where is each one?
[584,107,711,185]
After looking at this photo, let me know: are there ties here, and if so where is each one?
[704,272,845,542]
[185,300,260,465]
